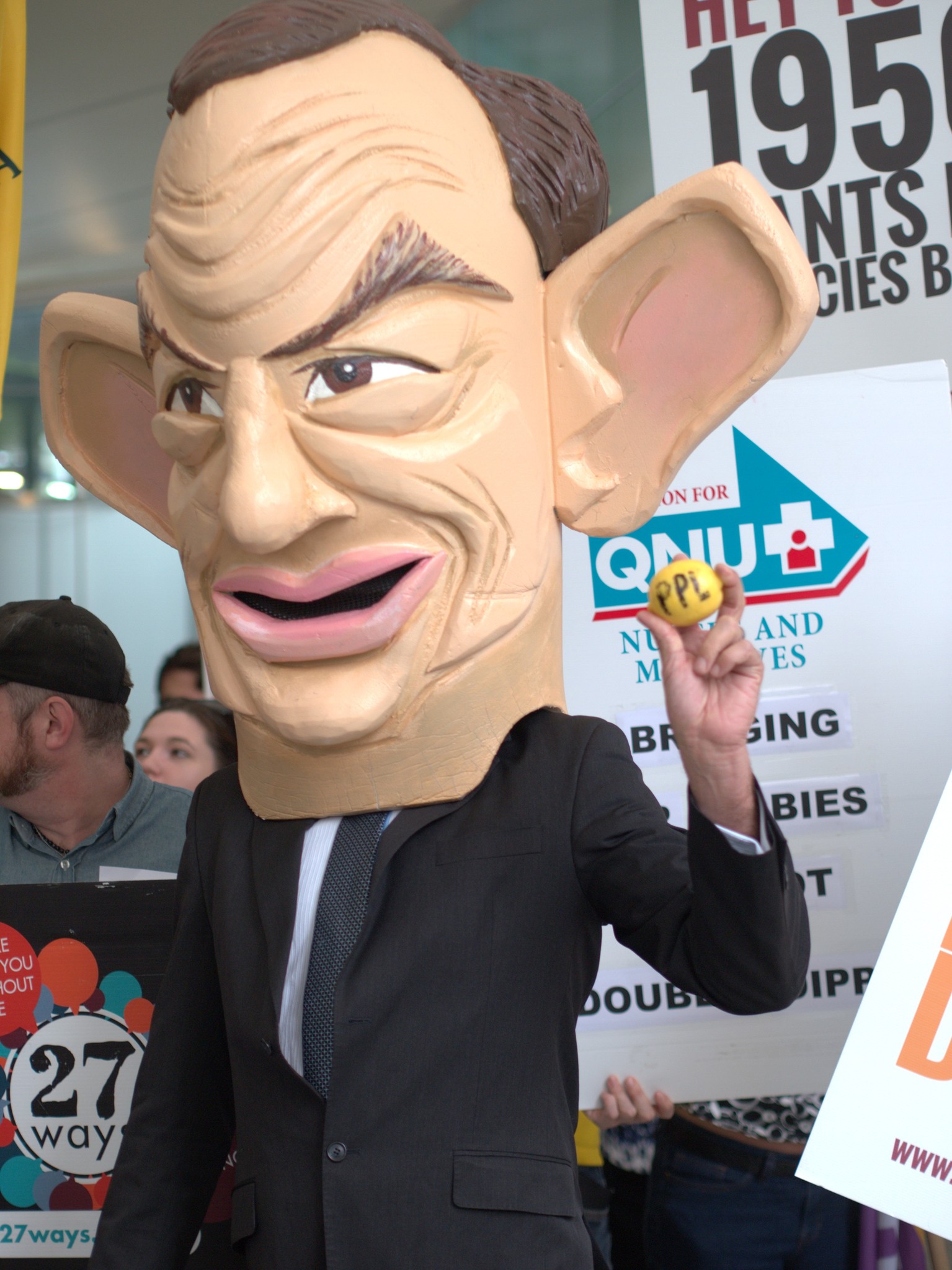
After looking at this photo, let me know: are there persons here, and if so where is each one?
[135,699,237,793]
[0,594,197,885]
[39,0,820,1270]
[158,645,203,703]
[583,1076,854,1270]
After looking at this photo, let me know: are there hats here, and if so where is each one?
[0,594,132,705]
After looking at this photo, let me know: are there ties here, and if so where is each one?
[299,803,388,1101]
